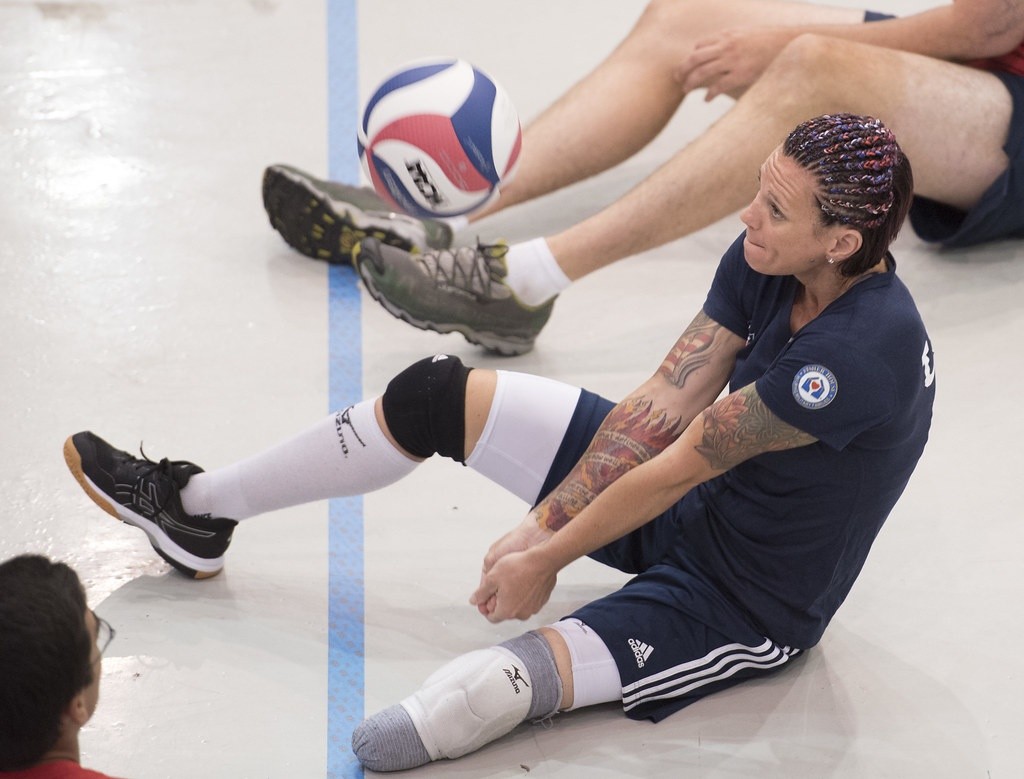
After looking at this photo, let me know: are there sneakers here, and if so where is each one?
[350,236,561,356]
[63,431,239,580]
[263,164,453,269]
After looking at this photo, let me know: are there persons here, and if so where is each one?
[0,554,122,779]
[64,111,936,773]
[262,0,1024,353]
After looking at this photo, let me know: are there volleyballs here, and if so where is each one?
[354,53,524,223]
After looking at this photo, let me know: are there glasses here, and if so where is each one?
[88,616,115,668]
